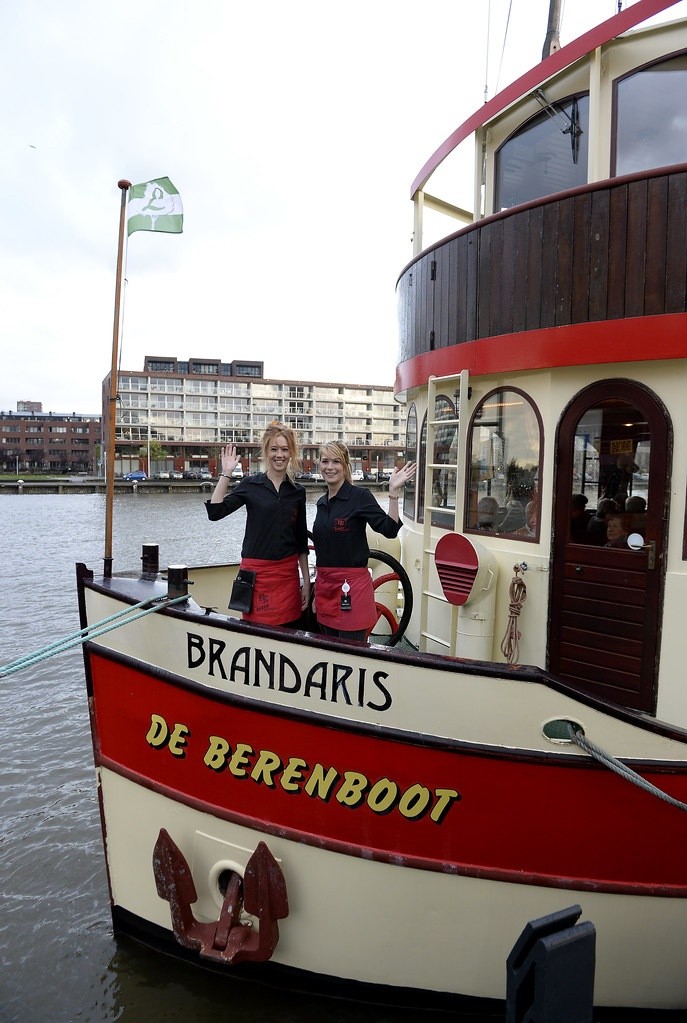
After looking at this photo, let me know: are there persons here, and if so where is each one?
[313,441,418,641]
[204,421,310,630]
[571,493,646,546]
[419,493,453,526]
[477,497,536,538]
[605,513,633,549]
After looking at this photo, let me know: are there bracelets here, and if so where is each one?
[388,495,399,499]
[219,474,232,480]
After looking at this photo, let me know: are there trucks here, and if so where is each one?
[231,463,244,479]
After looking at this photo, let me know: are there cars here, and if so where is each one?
[198,471,213,480]
[122,471,148,480]
[153,471,170,479]
[300,470,321,479]
[252,472,264,476]
[349,473,365,481]
[364,472,376,480]
[169,470,184,480]
[182,471,197,479]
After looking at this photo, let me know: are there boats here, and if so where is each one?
[73,3,687,1023]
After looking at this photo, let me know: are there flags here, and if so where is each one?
[128,177,183,236]
